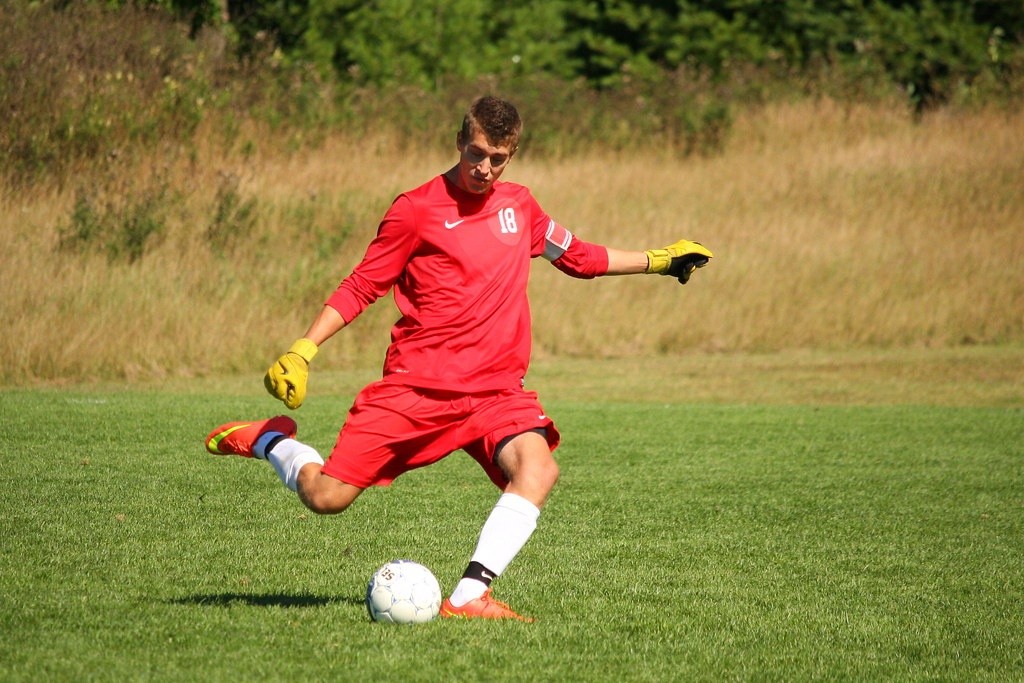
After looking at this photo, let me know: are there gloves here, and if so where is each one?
[264,338,319,409]
[642,239,714,284]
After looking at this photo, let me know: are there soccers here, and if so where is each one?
[367,559,443,626]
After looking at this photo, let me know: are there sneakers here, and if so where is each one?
[440,587,533,624]
[206,416,297,458]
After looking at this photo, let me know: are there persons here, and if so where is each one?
[205,96,713,623]
